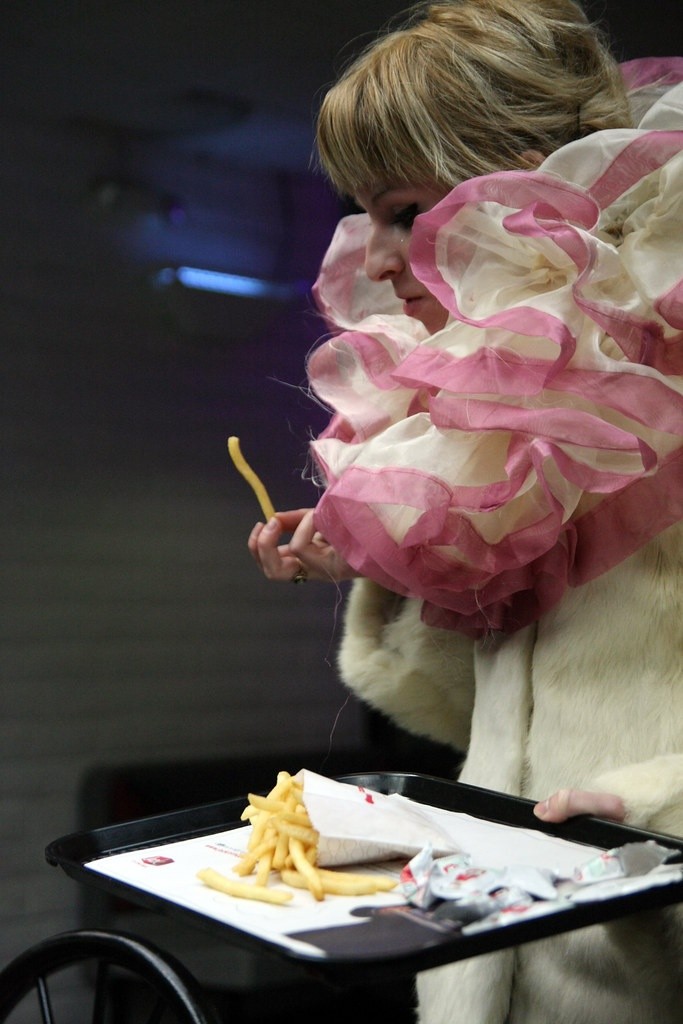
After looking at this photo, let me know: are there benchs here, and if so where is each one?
[74,745,413,1024]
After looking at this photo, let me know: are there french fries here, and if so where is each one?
[229,435,278,525]
[194,764,394,904]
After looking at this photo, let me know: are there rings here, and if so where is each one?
[292,569,307,585]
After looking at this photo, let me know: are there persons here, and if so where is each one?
[249,0,683,1024]
